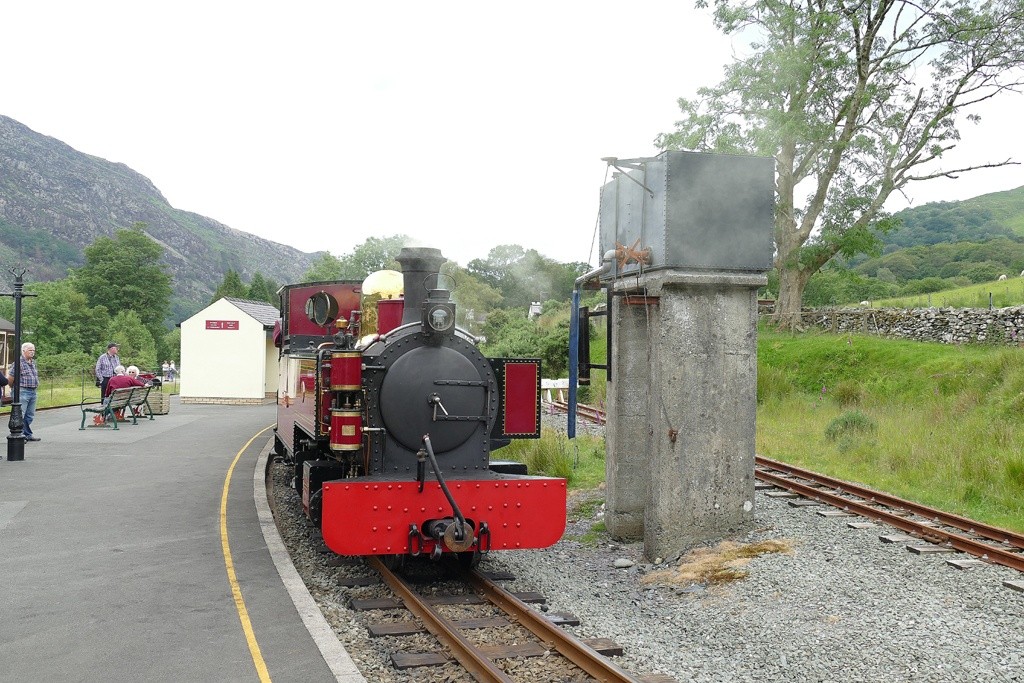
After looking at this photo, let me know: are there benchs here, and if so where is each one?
[80,386,154,430]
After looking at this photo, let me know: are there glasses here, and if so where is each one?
[129,372,135,373]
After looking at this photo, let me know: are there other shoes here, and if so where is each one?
[28,437,41,441]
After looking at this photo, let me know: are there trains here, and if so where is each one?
[275,247,568,585]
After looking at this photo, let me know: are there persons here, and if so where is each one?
[162,360,175,381]
[7,342,41,443]
[102,364,153,425]
[96,342,120,422]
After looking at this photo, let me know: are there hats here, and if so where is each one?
[108,342,122,348]
